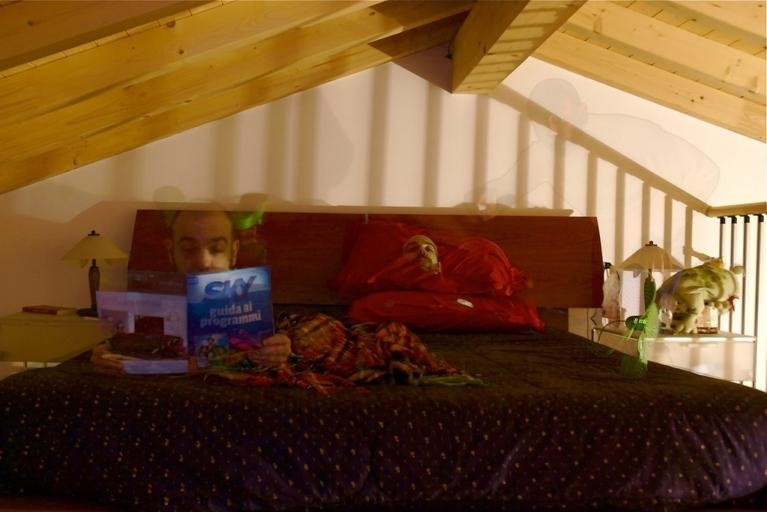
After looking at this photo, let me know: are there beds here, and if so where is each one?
[0,207,767,509]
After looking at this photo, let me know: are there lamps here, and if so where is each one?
[60,229,129,316]
[619,239,685,330]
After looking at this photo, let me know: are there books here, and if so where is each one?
[94,264,279,376]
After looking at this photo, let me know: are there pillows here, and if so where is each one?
[328,220,548,332]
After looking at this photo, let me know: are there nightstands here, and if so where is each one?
[1,308,118,363]
[591,322,756,387]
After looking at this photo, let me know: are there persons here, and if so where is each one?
[88,203,294,378]
[400,234,442,278]
[460,78,722,262]
